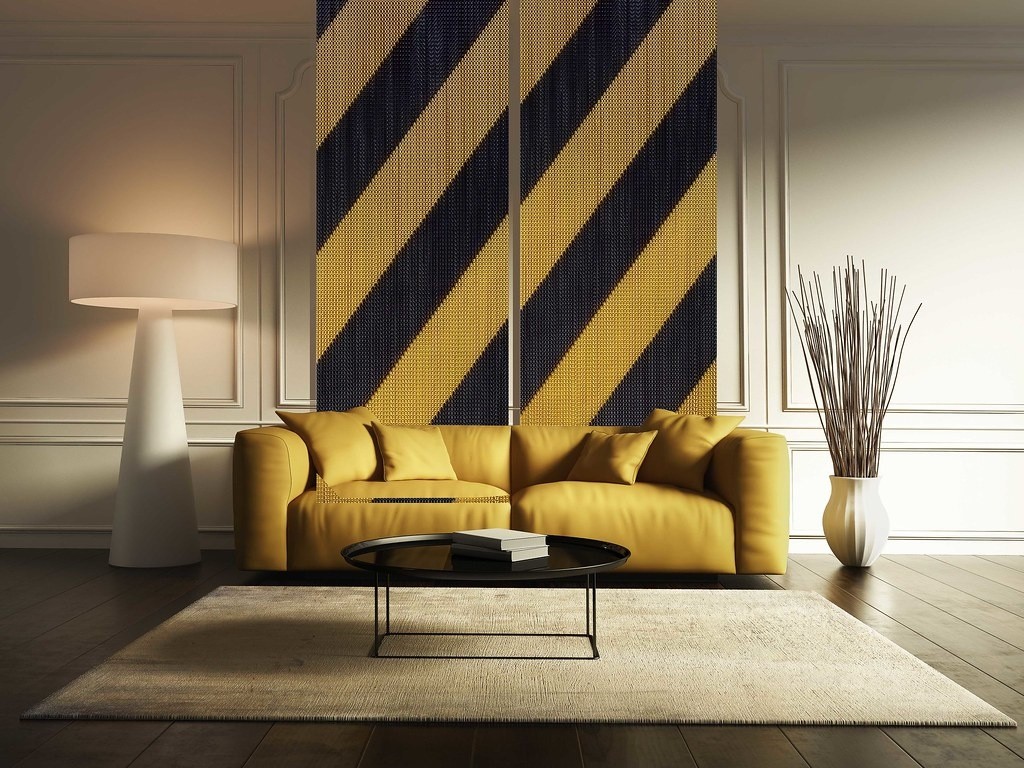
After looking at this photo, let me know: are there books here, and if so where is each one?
[450,543,549,562]
[454,557,550,570]
[452,528,547,551]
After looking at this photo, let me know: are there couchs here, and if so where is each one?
[233,424,790,583]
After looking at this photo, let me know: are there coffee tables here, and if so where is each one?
[340,533,631,660]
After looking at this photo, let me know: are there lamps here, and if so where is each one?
[69,232,238,569]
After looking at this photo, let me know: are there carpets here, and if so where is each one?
[17,586,1018,727]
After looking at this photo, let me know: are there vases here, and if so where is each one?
[822,475,890,567]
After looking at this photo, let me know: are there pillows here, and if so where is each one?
[274,407,382,487]
[371,421,458,482]
[635,408,746,493]
[566,430,658,486]
